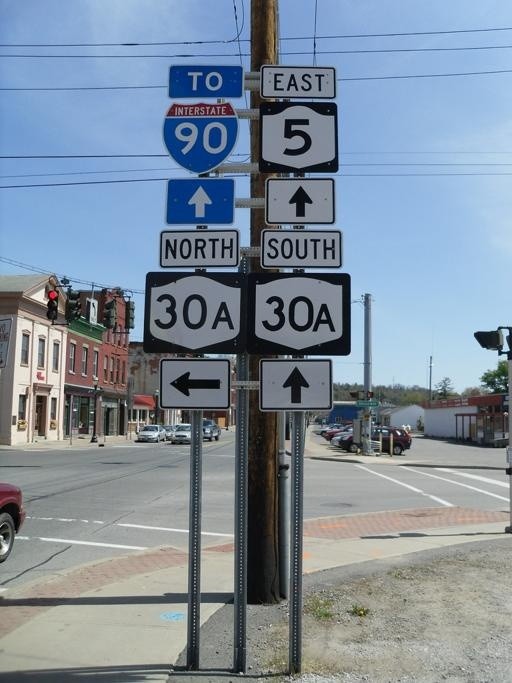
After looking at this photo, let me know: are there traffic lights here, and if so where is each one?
[65,288,80,322]
[45,289,59,321]
[103,300,116,328]
[366,390,373,399]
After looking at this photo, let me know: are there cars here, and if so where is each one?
[202,419,221,442]
[0,480,25,563]
[317,423,412,456]
[137,424,170,443]
[169,422,193,443]
